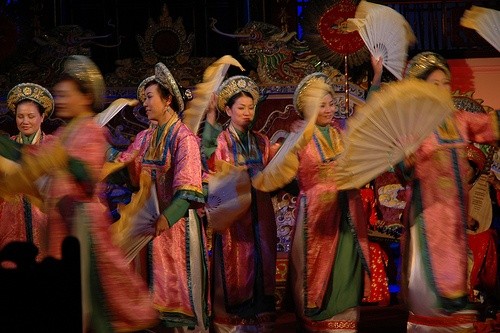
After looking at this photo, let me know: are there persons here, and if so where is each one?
[0,54,155,333]
[392,50,500,333]
[290,53,384,333]
[205,76,281,333]
[455,145,500,287]
[110,63,210,333]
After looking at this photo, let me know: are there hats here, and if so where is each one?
[7,83,54,117]
[60,54,103,110]
[404,51,451,81]
[138,74,154,102]
[293,73,335,119]
[155,63,183,112]
[217,76,259,114]
